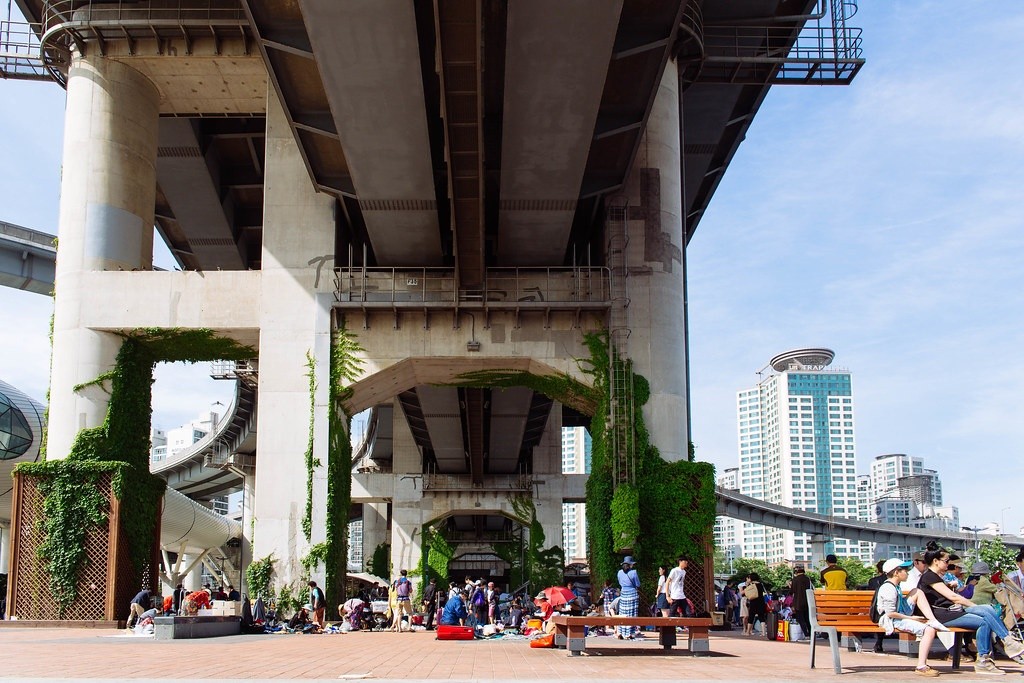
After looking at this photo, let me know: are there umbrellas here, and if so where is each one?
[533,584,576,607]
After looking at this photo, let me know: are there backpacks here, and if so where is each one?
[870,581,896,623]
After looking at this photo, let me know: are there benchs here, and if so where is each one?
[153,616,240,640]
[551,616,713,656]
[805,588,976,674]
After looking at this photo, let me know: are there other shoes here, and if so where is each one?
[742,632,749,636]
[408,628,415,632]
[947,653,973,662]
[759,634,765,637]
[915,667,939,676]
[676,627,685,632]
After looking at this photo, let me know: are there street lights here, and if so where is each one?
[1002,506,1012,542]
[962,525,989,563]
[726,550,733,575]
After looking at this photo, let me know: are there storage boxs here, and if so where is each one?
[710,611,726,625]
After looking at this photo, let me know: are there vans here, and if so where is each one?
[713,573,734,607]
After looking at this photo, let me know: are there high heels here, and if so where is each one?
[974,653,1006,674]
[1001,637,1024,659]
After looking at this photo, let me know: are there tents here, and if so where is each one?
[345,572,389,598]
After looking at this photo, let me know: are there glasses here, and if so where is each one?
[936,558,950,564]
[915,559,927,564]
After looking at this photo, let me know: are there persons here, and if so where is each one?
[819,554,848,592]
[181,583,212,615]
[215,587,228,600]
[395,569,416,633]
[665,556,688,631]
[654,564,671,618]
[162,595,173,617]
[227,586,240,601]
[617,556,642,640]
[138,606,163,626]
[289,608,309,628]
[308,582,325,630]
[421,574,621,639]
[174,584,184,613]
[865,541,1024,677]
[337,580,397,632]
[717,566,815,642]
[126,587,158,629]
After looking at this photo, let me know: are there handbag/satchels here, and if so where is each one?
[744,582,758,600]
[776,620,789,642]
[789,622,805,642]
[475,591,483,606]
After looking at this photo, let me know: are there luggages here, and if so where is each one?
[767,614,778,640]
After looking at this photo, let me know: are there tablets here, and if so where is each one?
[965,575,980,585]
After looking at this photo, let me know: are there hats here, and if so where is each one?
[534,592,548,600]
[474,579,482,585]
[302,605,312,611]
[882,558,914,575]
[914,551,926,560]
[949,560,967,568]
[971,563,993,573]
[620,555,637,565]
[338,604,345,617]
